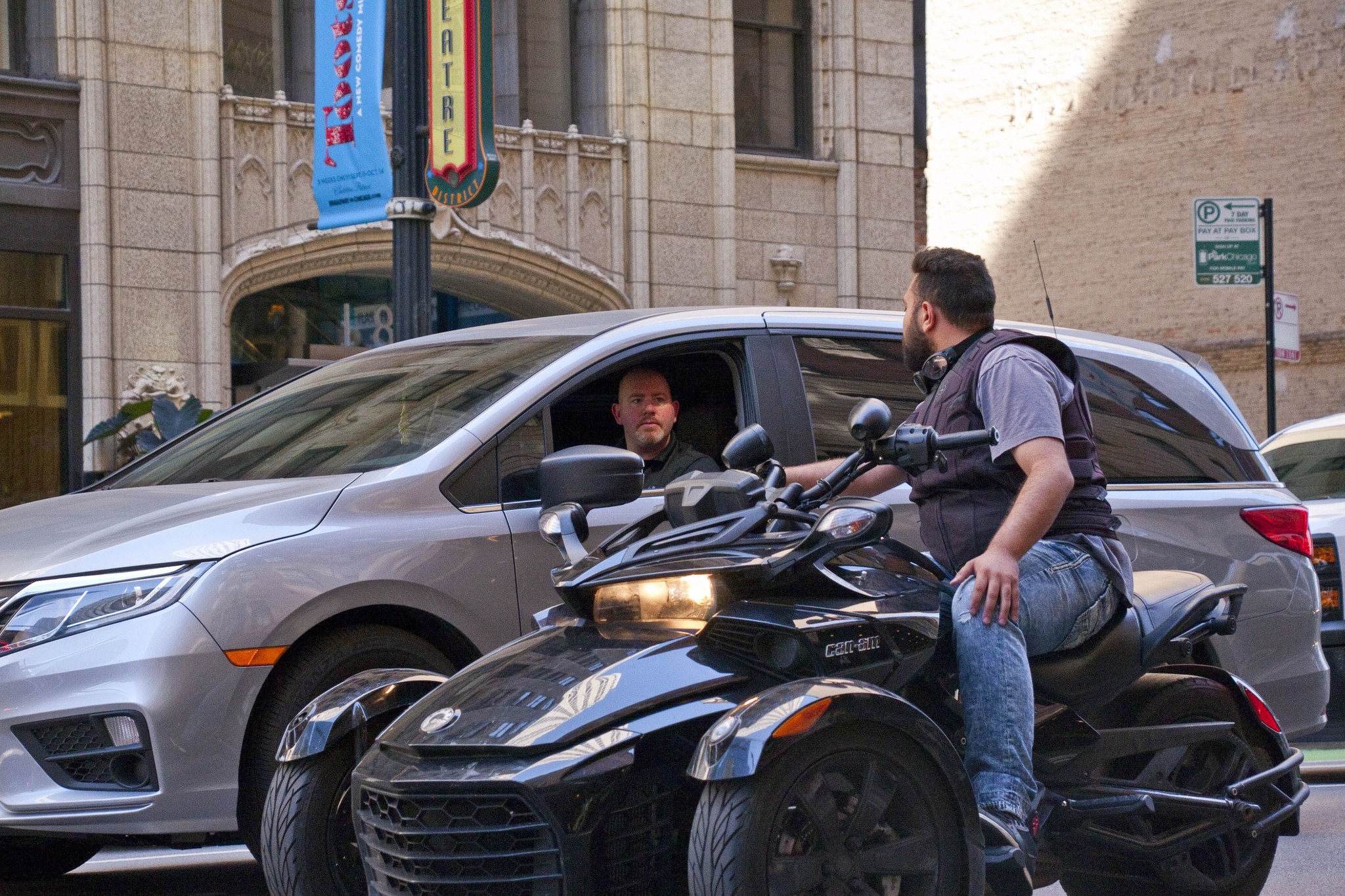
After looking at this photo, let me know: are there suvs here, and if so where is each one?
[0,308,1334,896]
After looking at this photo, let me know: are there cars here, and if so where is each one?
[1253,411,1345,742]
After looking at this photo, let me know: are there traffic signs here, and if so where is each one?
[1272,291,1303,364]
[1194,195,1264,286]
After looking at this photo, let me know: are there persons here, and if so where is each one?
[600,366,722,491]
[778,246,1134,896]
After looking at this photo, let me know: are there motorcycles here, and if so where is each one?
[259,397,1313,895]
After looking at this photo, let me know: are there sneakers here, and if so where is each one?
[979,808,1038,896]
